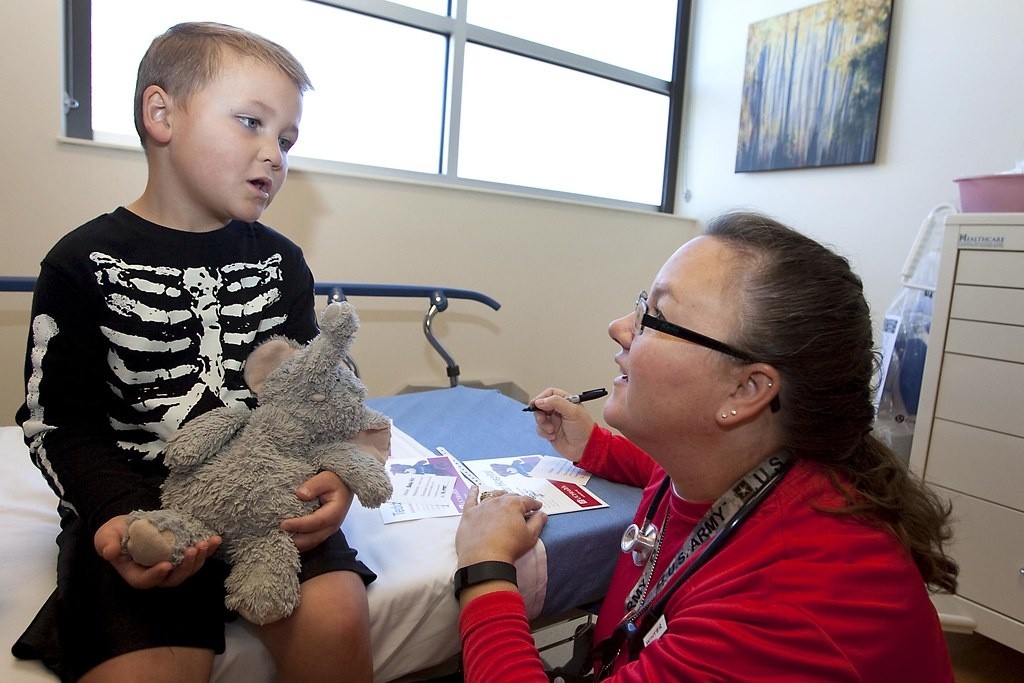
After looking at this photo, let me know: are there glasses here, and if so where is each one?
[630,290,783,415]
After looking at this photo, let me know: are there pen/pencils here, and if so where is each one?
[521,387,608,412]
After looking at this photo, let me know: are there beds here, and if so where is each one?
[0,275,651,682]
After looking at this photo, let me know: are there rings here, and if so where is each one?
[481,491,494,501]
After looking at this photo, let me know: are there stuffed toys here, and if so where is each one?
[120,302,393,618]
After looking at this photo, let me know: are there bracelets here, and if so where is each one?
[455,561,518,599]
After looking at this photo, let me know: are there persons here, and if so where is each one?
[451,211,959,683]
[12,22,392,682]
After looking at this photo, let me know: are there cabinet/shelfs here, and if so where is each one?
[903,210,1022,652]
